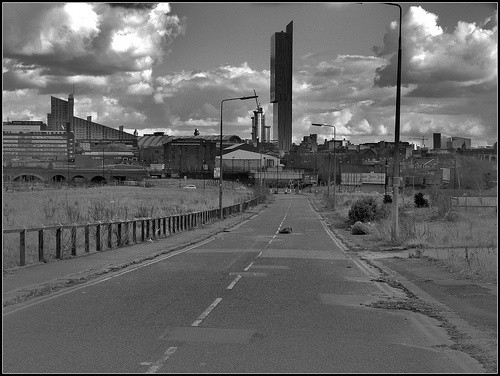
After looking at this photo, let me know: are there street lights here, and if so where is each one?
[311,123,337,211]
[217,96,260,220]
[355,3,403,248]
